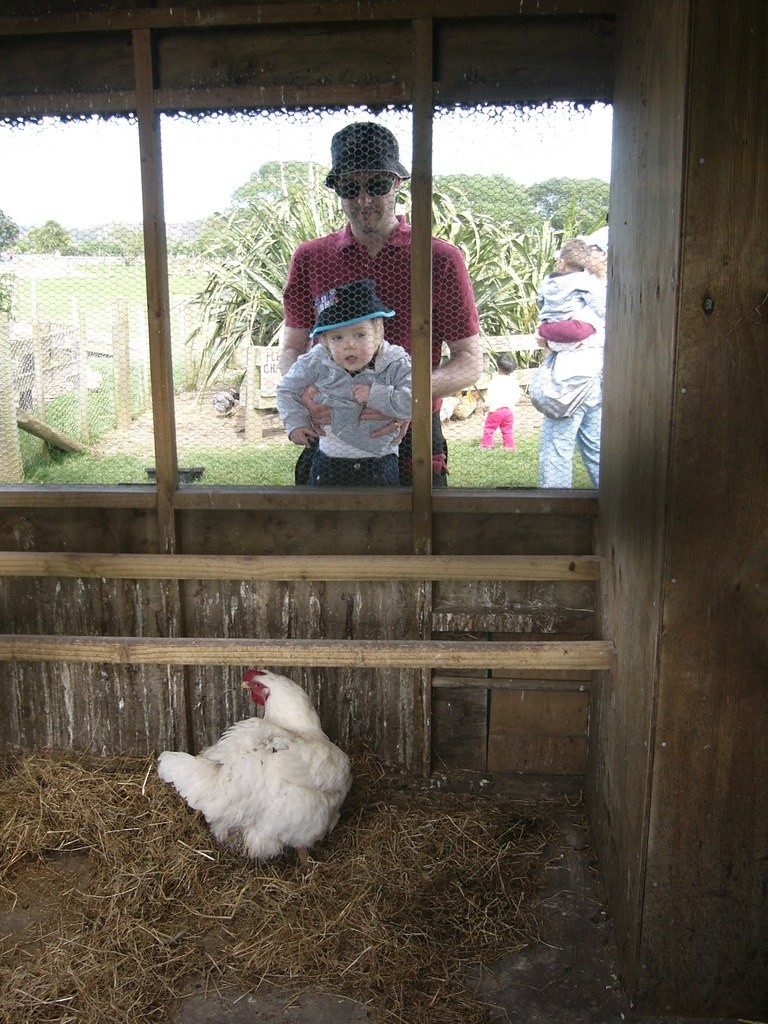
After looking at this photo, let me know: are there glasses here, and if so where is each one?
[333,174,396,199]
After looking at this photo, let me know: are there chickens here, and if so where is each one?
[156,669,354,882]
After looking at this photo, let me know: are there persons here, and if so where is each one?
[535,226,609,488]
[276,278,412,486]
[279,122,483,487]
[537,238,607,356]
[478,354,520,451]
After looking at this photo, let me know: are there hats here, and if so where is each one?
[309,278,396,338]
[576,226,609,252]
[325,122,411,189]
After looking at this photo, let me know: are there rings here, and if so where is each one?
[394,420,399,427]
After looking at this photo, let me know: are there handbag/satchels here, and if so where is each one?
[528,351,603,418]
